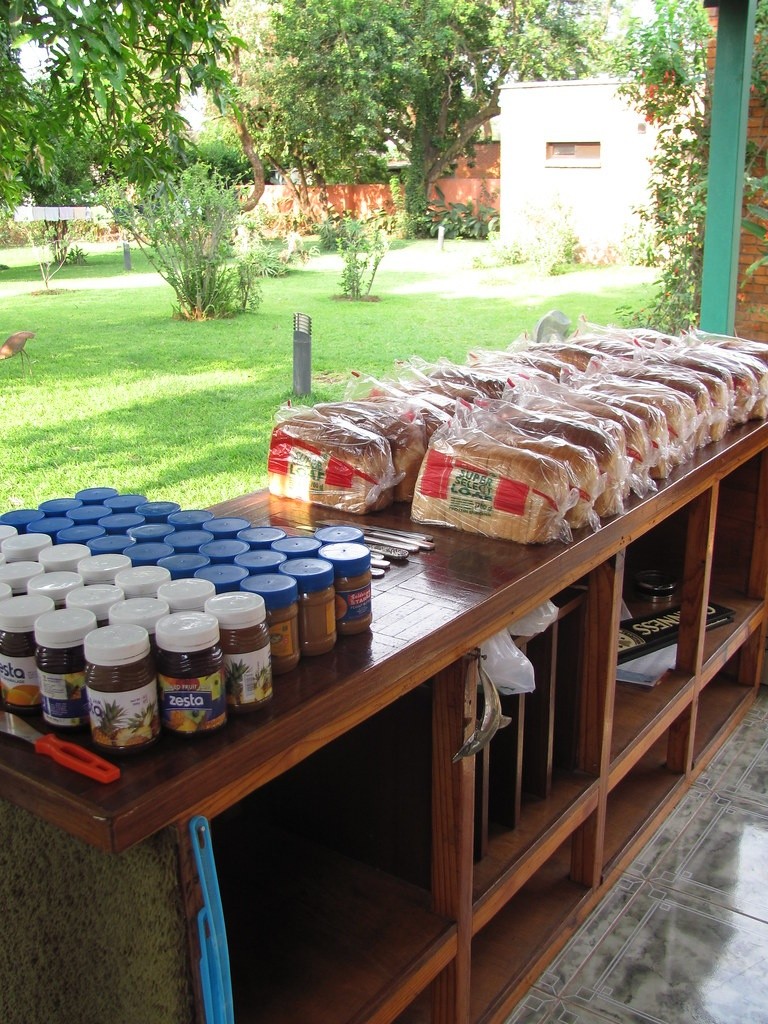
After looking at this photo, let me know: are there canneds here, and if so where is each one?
[0,488,372,755]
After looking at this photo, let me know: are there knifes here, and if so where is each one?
[270,518,436,577]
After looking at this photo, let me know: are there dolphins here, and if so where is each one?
[452,652,501,765]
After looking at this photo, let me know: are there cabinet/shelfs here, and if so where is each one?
[0,417,768,1024]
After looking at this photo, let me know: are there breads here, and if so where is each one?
[268,328,768,544]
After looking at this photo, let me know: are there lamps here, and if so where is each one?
[294,313,312,336]
